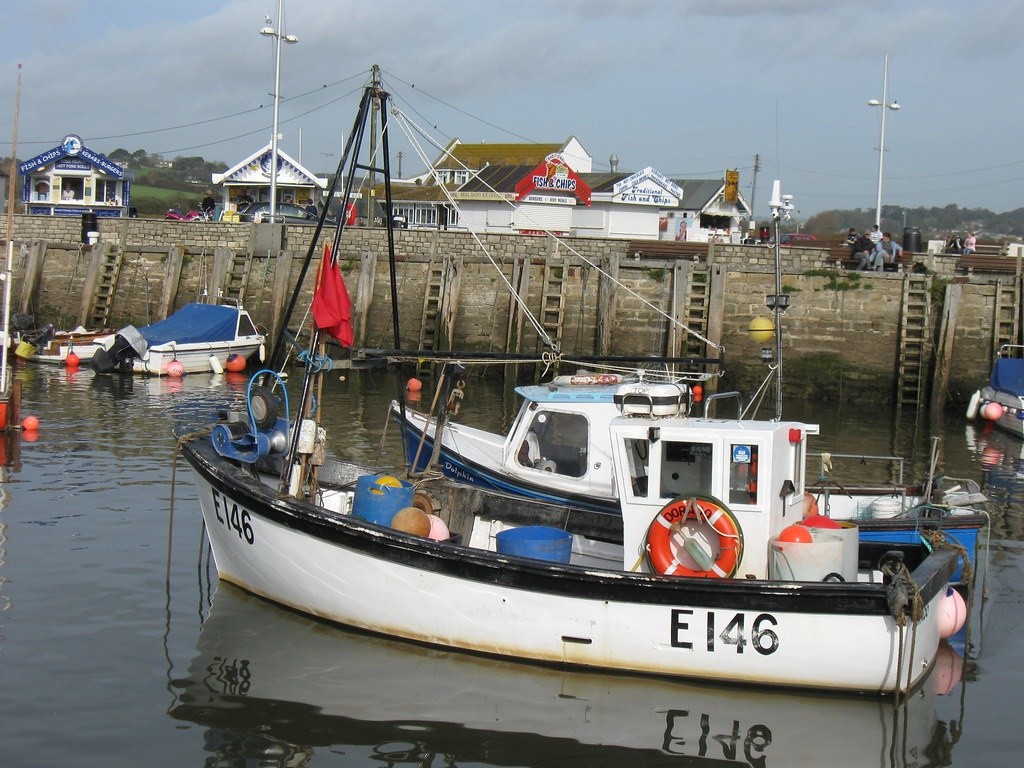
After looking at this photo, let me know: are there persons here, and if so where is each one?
[949,232,976,255]
[336,196,346,224]
[284,198,291,210]
[239,190,253,211]
[847,224,896,272]
[202,191,215,220]
[306,199,317,217]
[63,186,75,199]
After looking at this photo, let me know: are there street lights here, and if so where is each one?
[867,52,901,231]
[260,14,298,224]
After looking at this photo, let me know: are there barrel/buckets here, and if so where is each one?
[769,534,844,582]
[345,203,357,226]
[81,213,96,244]
[352,475,414,528]
[795,521,859,582]
[496,526,573,564]
[872,500,902,518]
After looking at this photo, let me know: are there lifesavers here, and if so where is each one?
[648,498,738,578]
[554,373,623,385]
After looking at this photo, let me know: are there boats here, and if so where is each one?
[387,369,989,586]
[966,344,1024,438]
[171,63,973,695]
[90,288,266,376]
[14,323,118,366]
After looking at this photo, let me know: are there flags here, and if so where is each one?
[312,244,354,348]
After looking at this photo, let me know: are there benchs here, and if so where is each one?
[624,239,1023,274]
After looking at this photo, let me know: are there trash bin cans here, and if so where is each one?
[744,239,756,245]
[392,215,408,229]
[80,212,98,244]
[128,206,137,218]
[903,226,922,253]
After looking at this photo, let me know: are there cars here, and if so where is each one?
[239,200,341,225]
[766,232,816,247]
